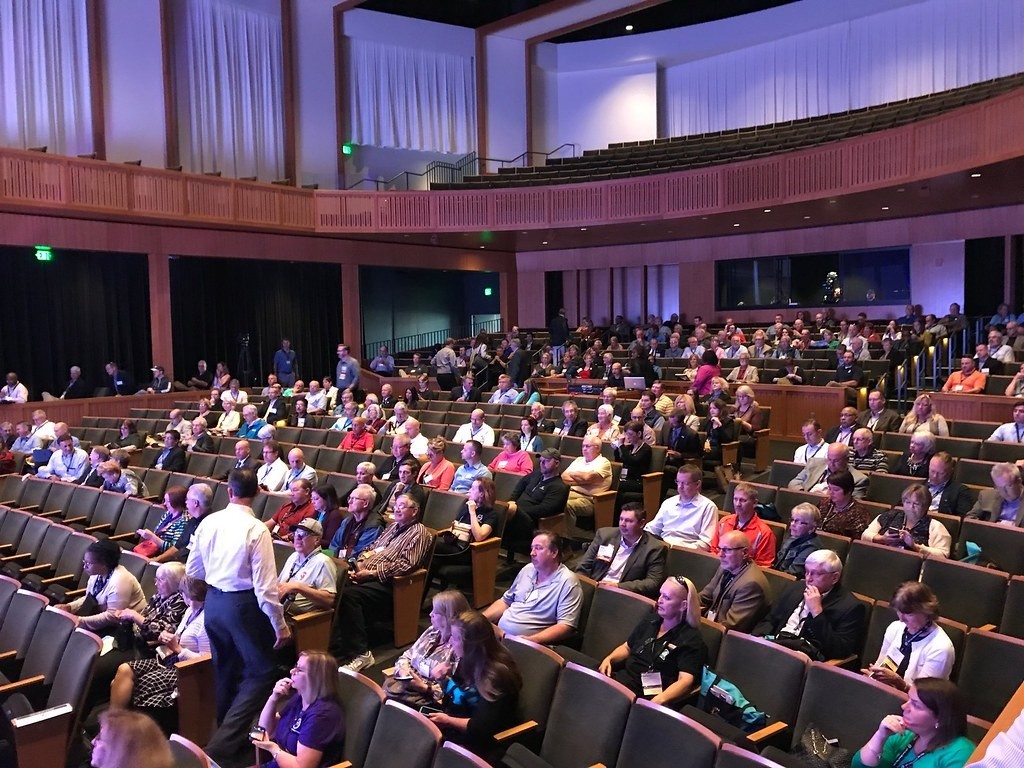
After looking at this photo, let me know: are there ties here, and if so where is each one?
[237,461,241,468]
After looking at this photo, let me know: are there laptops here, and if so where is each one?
[624,376,651,391]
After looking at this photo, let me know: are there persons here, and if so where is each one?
[89,710,174,767]
[251,648,344,768]
[851,677,977,768]
[180,469,292,768]
[0,302,1023,767]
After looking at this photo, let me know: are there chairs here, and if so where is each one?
[430,73,1024,192]
[0,315,1024,768]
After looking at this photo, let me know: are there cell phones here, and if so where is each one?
[419,706,444,717]
[394,675,413,681]
[250,725,265,741]
[889,528,900,538]
[442,531,457,539]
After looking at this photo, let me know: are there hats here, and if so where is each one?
[534,447,562,461]
[296,518,323,537]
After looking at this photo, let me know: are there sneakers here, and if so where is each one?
[341,650,376,672]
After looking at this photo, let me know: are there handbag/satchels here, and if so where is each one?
[801,721,853,768]
[133,539,159,559]
[451,520,472,542]
[951,540,983,565]
[765,630,821,662]
[700,666,770,731]
[381,666,438,708]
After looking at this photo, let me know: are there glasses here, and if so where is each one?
[347,440,439,528]
[82,560,105,566]
[294,664,309,675]
[994,479,1018,490]
[804,571,836,578]
[154,577,172,582]
[294,531,313,537]
[674,476,808,594]
